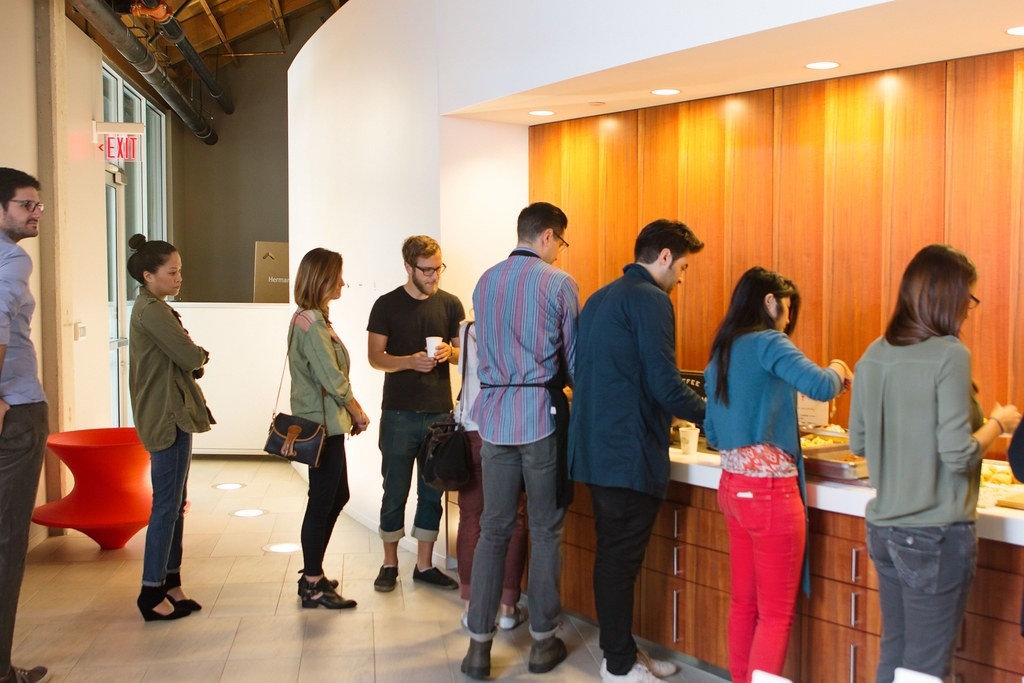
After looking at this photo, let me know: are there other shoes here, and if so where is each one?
[499,605,528,630]
[412,563,459,590]
[374,565,398,592]
[460,611,468,627]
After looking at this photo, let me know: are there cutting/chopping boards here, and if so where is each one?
[997,493,1024,510]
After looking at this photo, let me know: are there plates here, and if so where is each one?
[999,432,1011,437]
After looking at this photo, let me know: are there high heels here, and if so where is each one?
[297,575,357,609]
[137,572,202,622]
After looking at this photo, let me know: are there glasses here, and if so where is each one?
[10,199,45,212]
[553,230,569,253]
[413,263,447,276]
[967,294,981,309]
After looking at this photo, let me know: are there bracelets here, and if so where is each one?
[830,362,847,379]
[988,416,1004,434]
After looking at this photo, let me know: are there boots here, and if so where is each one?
[529,638,568,673]
[461,637,493,677]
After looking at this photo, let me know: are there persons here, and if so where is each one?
[707,267,854,682]
[573,220,705,683]
[125,234,217,620]
[453,320,529,630]
[849,244,1024,683]
[0,166,49,683]
[284,249,371,609]
[459,203,582,678]
[366,235,470,593]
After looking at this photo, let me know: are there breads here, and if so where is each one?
[979,464,1018,488]
[800,424,848,449]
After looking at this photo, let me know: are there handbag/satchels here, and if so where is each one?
[263,412,327,469]
[418,417,474,491]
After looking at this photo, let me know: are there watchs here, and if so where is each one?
[450,347,456,359]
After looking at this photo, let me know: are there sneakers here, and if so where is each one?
[0,666,48,683]
[600,645,676,683]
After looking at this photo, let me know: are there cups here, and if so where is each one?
[426,336,442,358]
[680,427,700,455]
